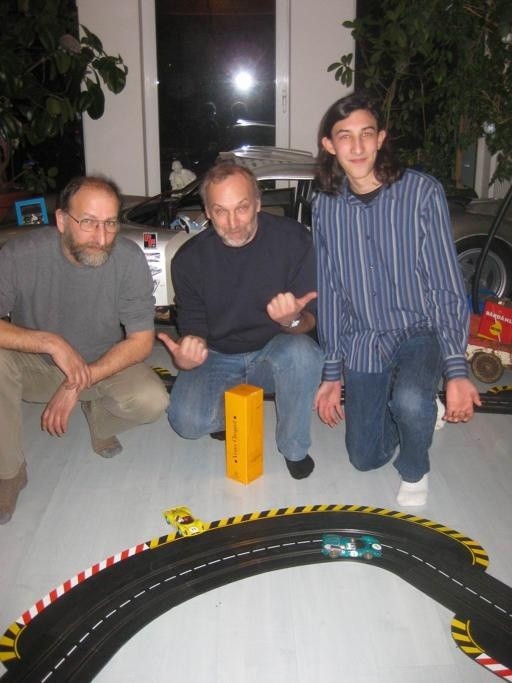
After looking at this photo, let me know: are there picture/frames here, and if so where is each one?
[16,197,50,226]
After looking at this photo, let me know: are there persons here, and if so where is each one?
[222,100,257,143]
[188,103,219,143]
[168,159,197,190]
[1,174,169,525]
[312,92,484,506]
[156,161,321,482]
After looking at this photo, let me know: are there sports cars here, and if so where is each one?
[119,143,512,322]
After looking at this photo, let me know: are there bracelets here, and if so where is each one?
[279,313,305,327]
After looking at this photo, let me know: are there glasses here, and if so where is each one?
[63,209,124,234]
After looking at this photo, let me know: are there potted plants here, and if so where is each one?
[0,0,131,223]
